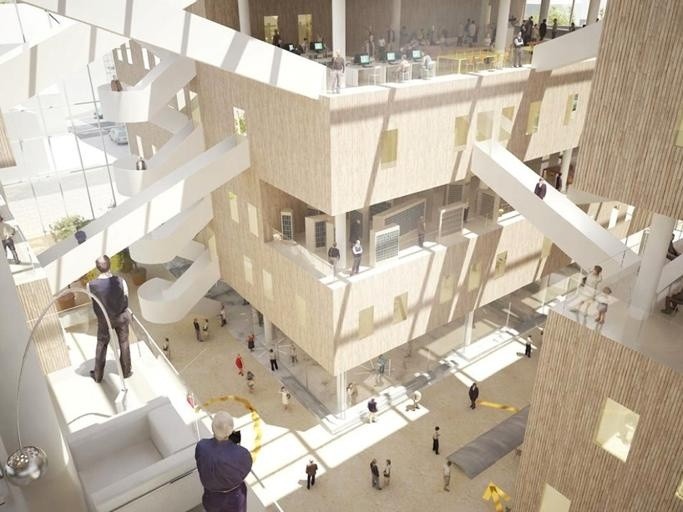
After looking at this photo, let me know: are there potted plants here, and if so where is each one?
[115,248,146,286]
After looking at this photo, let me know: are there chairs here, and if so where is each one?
[367,56,437,85]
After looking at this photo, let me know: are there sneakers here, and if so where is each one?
[123,370,134,378]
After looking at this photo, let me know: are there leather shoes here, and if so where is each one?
[90,370,100,383]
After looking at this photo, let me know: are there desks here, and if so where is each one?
[436,51,504,76]
[345,64,385,87]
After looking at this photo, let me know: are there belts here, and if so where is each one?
[210,483,242,494]
